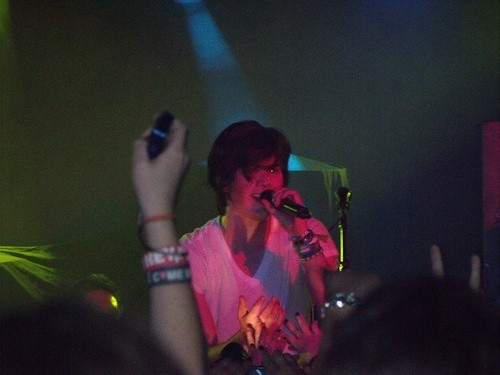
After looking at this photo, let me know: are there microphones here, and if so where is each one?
[260,190,312,219]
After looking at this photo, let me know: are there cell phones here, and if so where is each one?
[146,112,175,160]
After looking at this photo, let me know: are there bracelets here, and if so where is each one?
[146,267,191,288]
[139,213,176,233]
[143,244,189,269]
[290,227,323,262]
[321,291,363,322]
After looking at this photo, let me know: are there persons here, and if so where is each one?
[207,245,500,375]
[179,120,339,355]
[0,110,207,375]
